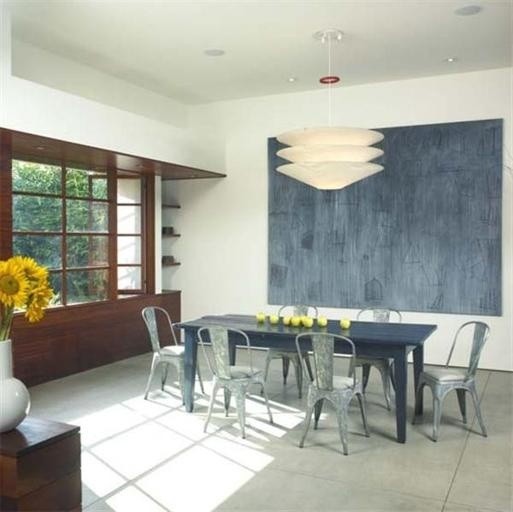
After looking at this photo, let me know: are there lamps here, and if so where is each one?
[411,322,491,442]
[347,305,402,411]
[258,304,319,399]
[197,326,273,438]
[143,306,205,405]
[294,333,370,456]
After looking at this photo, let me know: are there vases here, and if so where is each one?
[0,257,55,343]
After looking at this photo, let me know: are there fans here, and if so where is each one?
[267,118,502,318]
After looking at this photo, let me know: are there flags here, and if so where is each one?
[0,339,30,434]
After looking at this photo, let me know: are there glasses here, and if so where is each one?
[275,28,385,190]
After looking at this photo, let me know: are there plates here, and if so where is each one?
[161,203,180,266]
[0,419,87,512]
